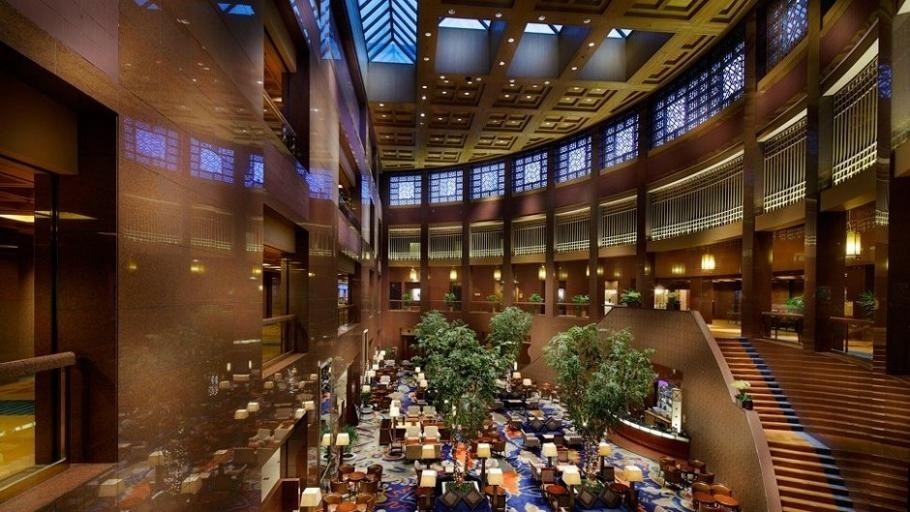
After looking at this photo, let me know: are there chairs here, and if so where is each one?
[227,464,248,492]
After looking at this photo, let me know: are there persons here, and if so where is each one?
[321,392,330,414]
[403,393,424,411]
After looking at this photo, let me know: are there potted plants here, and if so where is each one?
[730,380,751,407]
[401,293,589,317]
[619,289,642,307]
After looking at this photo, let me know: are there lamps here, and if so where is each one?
[98,449,229,503]
[190,255,262,279]
[597,250,715,276]
[220,373,318,431]
[845,219,862,256]
[300,487,322,507]
